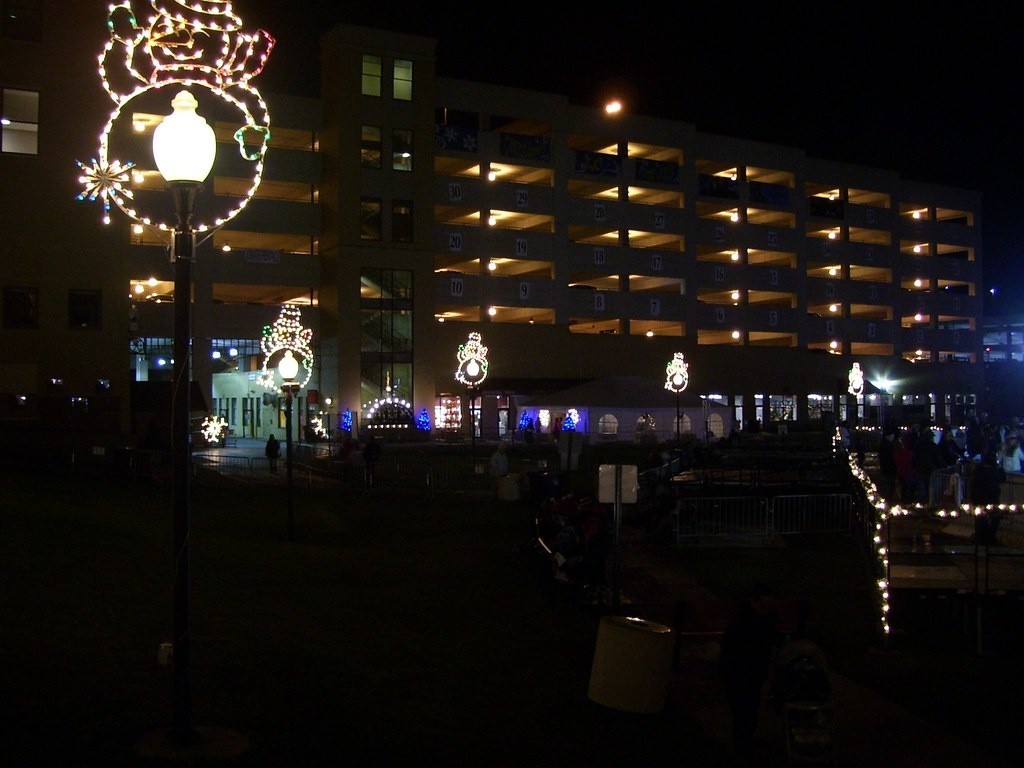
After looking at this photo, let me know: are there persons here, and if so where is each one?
[527,418,535,432]
[552,490,610,592]
[727,429,740,448]
[718,583,786,768]
[491,442,509,503]
[535,417,541,433]
[265,434,280,474]
[362,436,383,487]
[550,417,564,444]
[839,424,1024,546]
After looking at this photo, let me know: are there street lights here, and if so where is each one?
[148,88,218,584]
[673,371,683,448]
[464,359,479,448]
[277,351,302,466]
